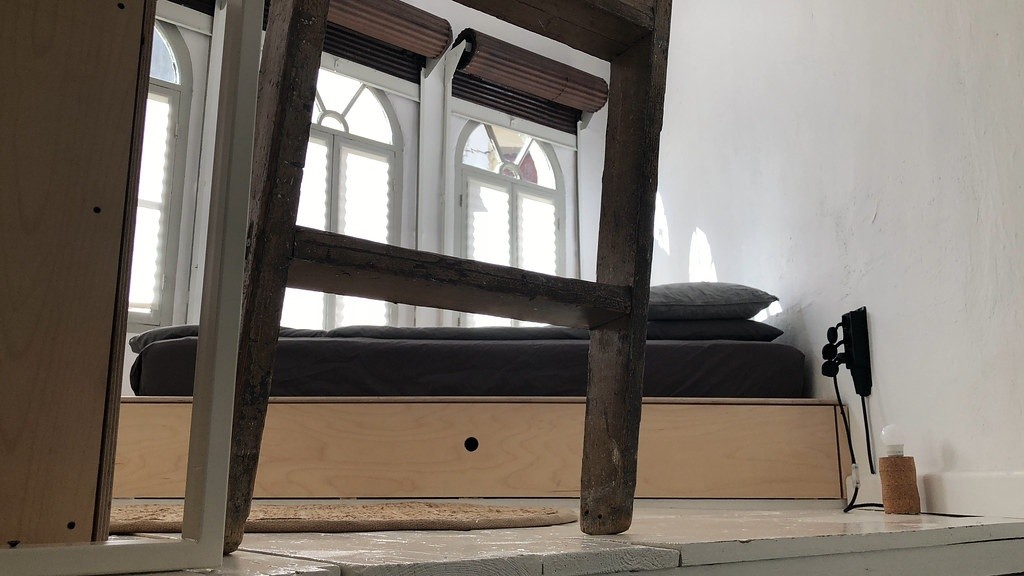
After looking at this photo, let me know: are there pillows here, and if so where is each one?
[648,320,785,340]
[647,282,779,320]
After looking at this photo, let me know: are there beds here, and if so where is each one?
[110,323,856,502]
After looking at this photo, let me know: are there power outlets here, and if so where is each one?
[841,305,872,398]
